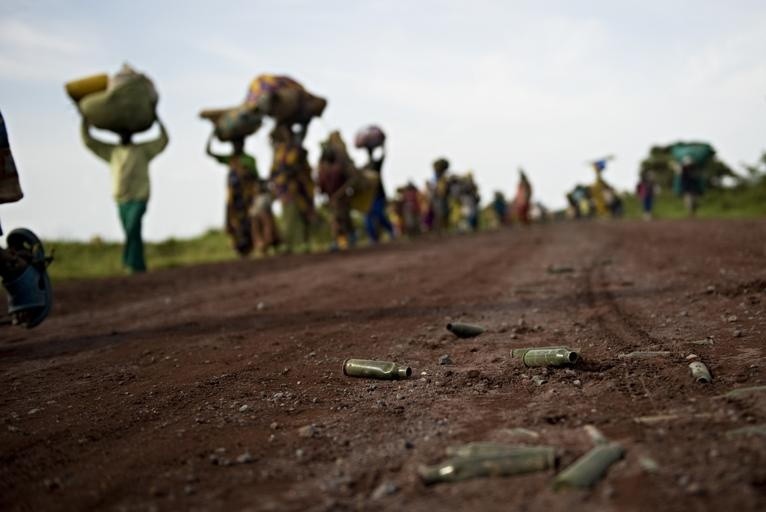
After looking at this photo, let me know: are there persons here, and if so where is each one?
[567,146,729,216]
[267,109,325,255]
[244,179,286,261]
[315,125,534,250]
[207,128,260,260]
[0,108,53,329]
[81,108,170,275]
